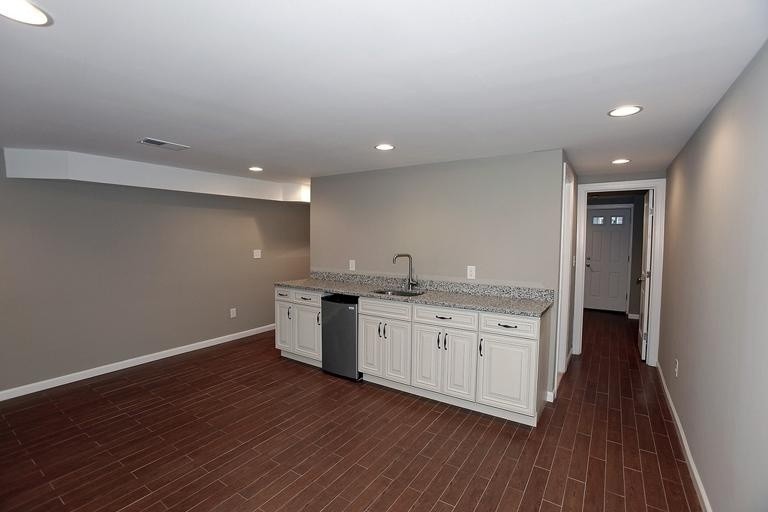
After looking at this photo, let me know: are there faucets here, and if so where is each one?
[393,253,418,289]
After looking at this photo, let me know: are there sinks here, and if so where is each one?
[374,290,422,296]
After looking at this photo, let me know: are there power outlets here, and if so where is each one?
[230,308,237,318]
[467,266,476,279]
[349,260,356,271]
[253,249,261,258]
[673,359,678,377]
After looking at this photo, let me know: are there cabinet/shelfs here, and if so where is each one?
[358,296,413,394]
[275,285,293,359]
[476,311,549,428]
[411,303,479,412]
[292,288,333,369]
